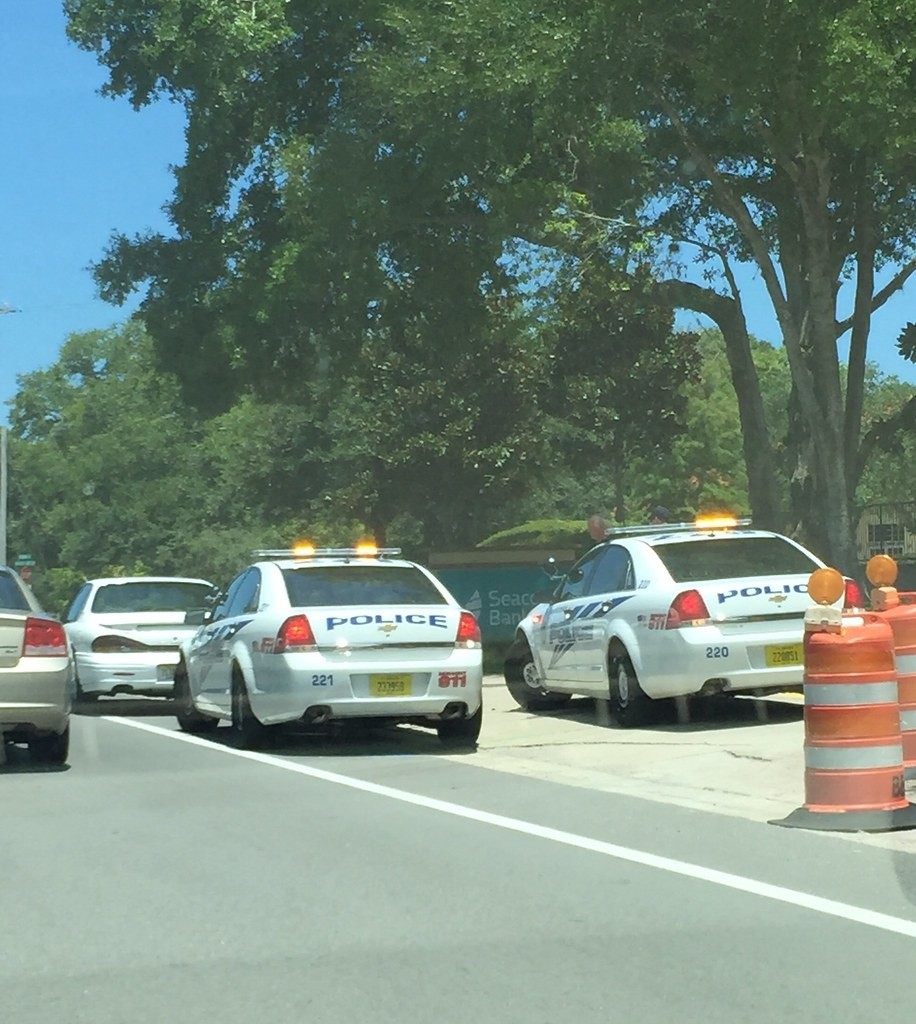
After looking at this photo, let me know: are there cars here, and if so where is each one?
[58,576,223,700]
[503,530,864,729]
[173,558,483,752]
[0,565,74,765]
